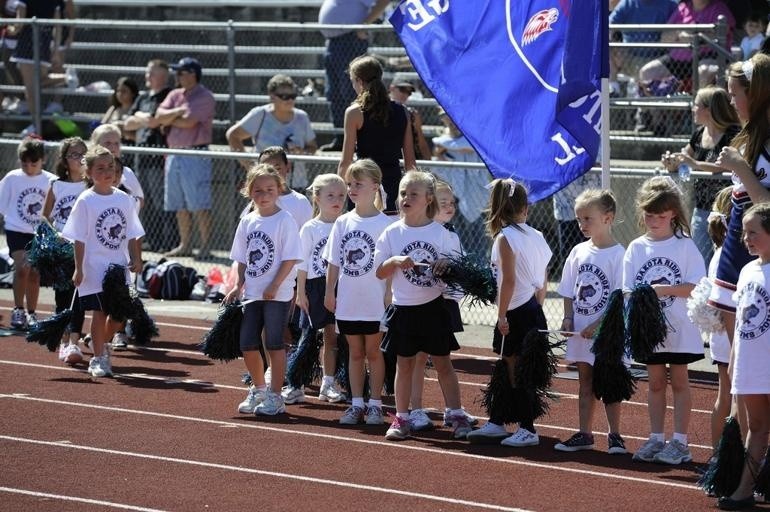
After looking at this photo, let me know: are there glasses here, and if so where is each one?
[395,86,411,96]
[270,89,298,100]
[64,150,86,160]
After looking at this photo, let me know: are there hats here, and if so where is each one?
[390,74,416,91]
[165,57,202,71]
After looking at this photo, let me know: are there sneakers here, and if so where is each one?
[64,67,78,88]
[1,96,64,116]
[58,331,129,378]
[318,382,347,403]
[385,408,540,446]
[238,364,312,416]
[635,111,670,138]
[320,137,343,151]
[339,391,384,426]
[141,241,211,260]
[632,436,692,465]
[9,308,40,329]
[704,469,770,510]
[608,432,626,454]
[554,432,594,452]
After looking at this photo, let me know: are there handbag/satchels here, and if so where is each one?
[134,258,204,300]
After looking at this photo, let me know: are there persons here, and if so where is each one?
[1,0,215,377]
[221,0,770,511]
